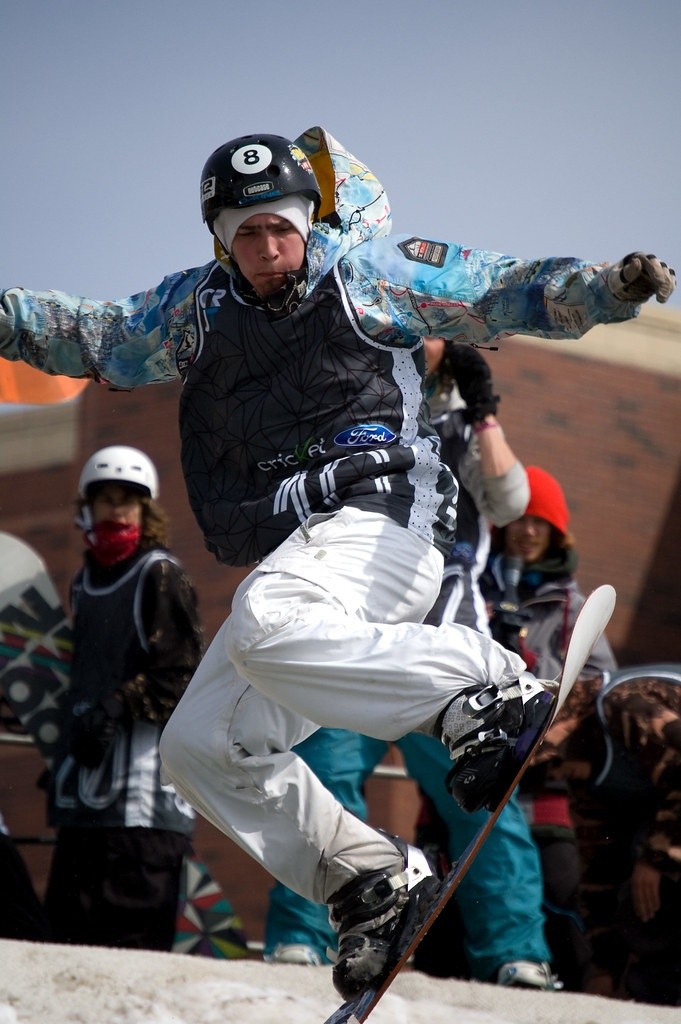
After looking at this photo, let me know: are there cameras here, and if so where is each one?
[490,602,532,656]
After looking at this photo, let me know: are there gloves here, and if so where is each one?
[609,251,677,305]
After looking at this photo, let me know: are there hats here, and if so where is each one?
[523,463,570,542]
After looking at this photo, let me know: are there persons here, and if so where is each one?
[40,445,207,951]
[0,128,677,992]
[417,464,611,990]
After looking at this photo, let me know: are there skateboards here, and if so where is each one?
[323,582,621,1022]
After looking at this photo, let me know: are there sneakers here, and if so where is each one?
[495,959,560,993]
[276,942,320,965]
[434,679,550,812]
[327,826,439,993]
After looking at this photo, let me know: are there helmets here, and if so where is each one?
[200,134,321,231]
[78,445,160,500]
